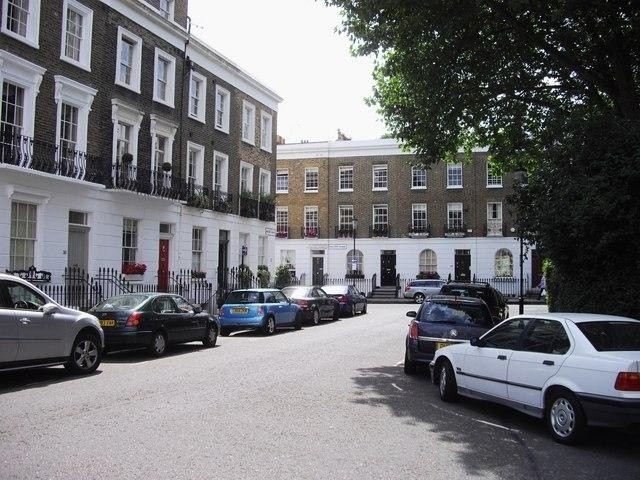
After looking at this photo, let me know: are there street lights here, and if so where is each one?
[518,182,530,313]
[351,216,359,287]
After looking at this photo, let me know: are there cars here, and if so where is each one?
[87,289,220,355]
[217,287,303,335]
[426,312,639,445]
[0,272,106,377]
[403,294,494,374]
[282,284,340,325]
[320,283,368,317]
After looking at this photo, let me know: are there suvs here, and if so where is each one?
[438,279,510,325]
[403,280,448,304]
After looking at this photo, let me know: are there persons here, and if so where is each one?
[535,275,547,300]
